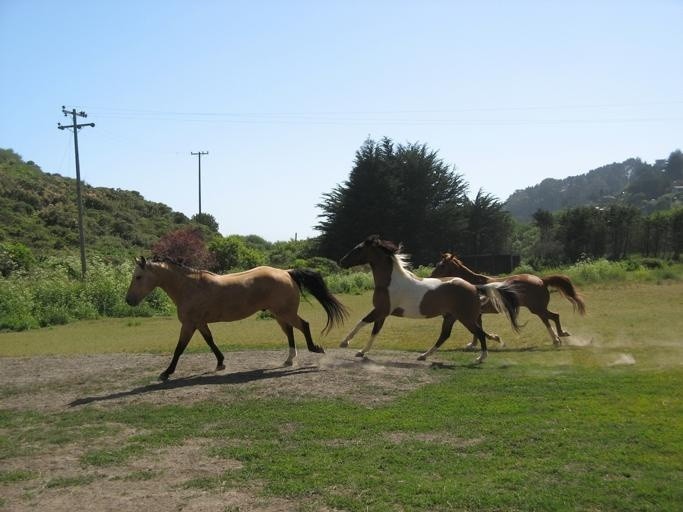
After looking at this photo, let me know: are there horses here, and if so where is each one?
[124,255,349,381]
[428,248,587,349]
[339,234,489,363]
